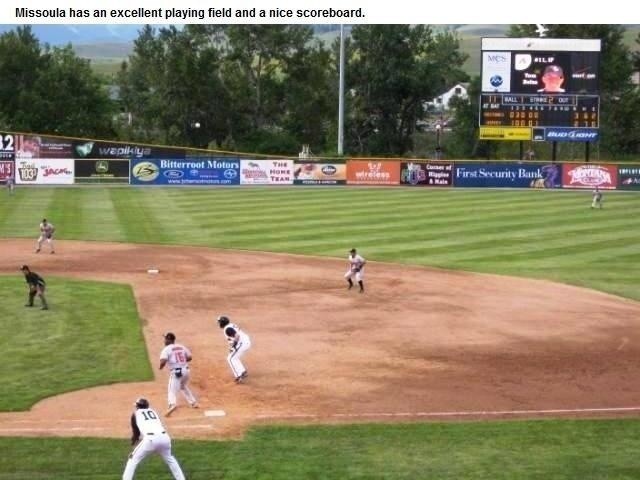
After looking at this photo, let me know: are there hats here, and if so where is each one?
[136,399,148,409]
[217,316,229,324]
[162,333,175,340]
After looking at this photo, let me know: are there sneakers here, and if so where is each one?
[165,403,176,416]
[234,372,248,383]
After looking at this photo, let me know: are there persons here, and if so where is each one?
[6,171,15,196]
[34,218,55,255]
[216,316,252,383]
[120,397,186,480]
[19,264,48,311]
[590,184,602,210]
[158,331,202,417]
[342,246,366,294]
[536,65,566,92]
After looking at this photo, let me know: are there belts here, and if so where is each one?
[147,431,165,435]
